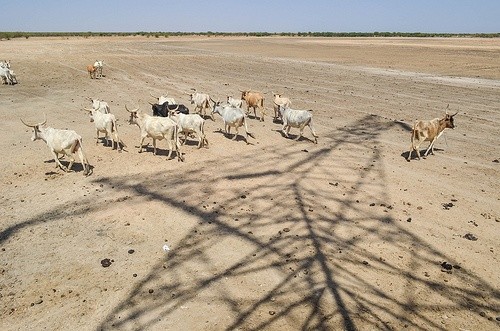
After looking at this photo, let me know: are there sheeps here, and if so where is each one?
[407,103,459,162]
[87,65,98,80]
[0,59,18,85]
[19,112,93,176]
[93,59,104,79]
[84,88,319,162]
